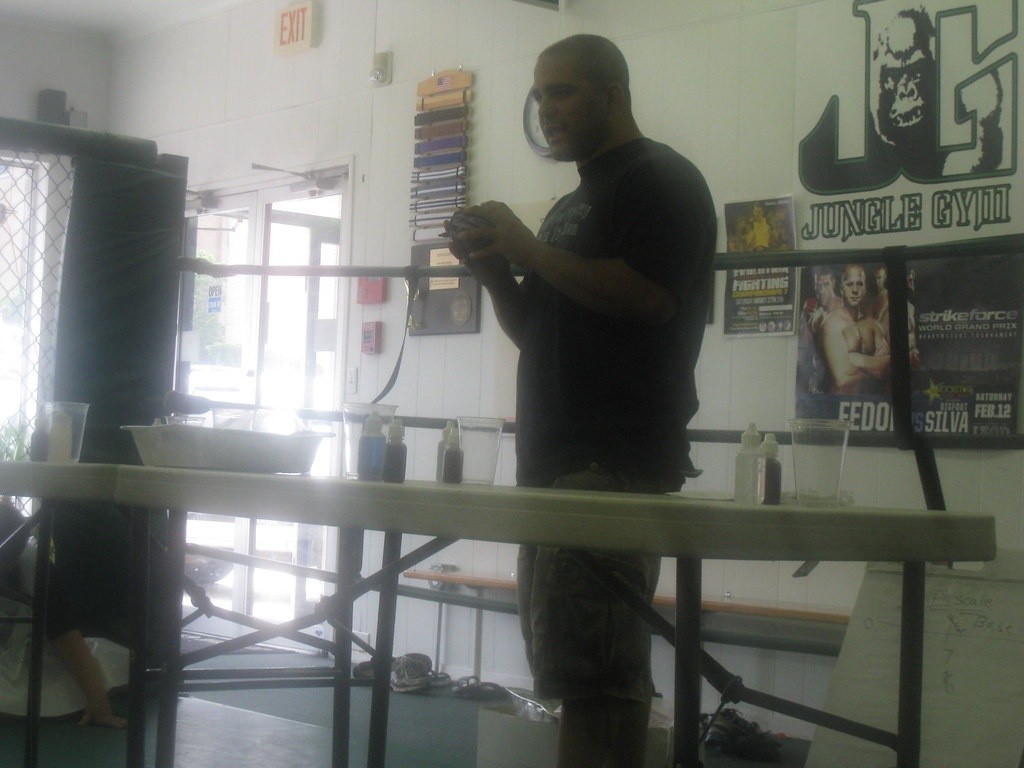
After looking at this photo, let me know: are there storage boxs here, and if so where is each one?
[120,420,322,473]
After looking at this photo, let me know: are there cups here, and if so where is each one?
[790,420,852,508]
[165,416,205,427]
[45,402,89,462]
[213,408,253,431]
[341,403,398,480]
[458,417,504,485]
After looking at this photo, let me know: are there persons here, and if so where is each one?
[746,203,775,250]
[0,494,216,730]
[436,33,720,768]
[799,260,923,402]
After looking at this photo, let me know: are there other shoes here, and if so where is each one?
[430,671,451,686]
[451,676,510,700]
[353,654,432,677]
[390,656,431,692]
[705,708,783,761]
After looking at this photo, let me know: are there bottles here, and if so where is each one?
[383,419,407,483]
[761,434,781,506]
[358,411,386,483]
[437,421,457,484]
[734,423,765,505]
[30,415,48,462]
[443,429,463,485]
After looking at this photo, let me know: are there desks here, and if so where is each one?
[0,460,150,768]
[112,463,997,767]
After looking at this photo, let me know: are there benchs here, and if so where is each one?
[403,569,851,627]
